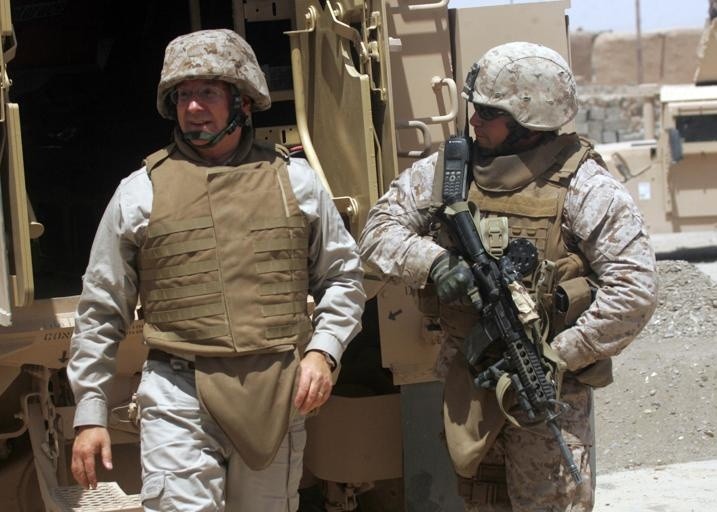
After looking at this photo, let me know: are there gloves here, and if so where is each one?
[429,252,483,311]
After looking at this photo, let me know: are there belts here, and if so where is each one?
[148,350,195,372]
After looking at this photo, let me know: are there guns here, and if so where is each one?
[448,202,584,486]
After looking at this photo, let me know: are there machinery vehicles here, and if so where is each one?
[587,14,716,265]
[0,0,600,510]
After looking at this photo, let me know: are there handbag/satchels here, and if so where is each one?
[442,336,513,477]
[195,350,301,474]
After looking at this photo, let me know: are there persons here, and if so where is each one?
[357,41,658,511]
[66,28,366,512]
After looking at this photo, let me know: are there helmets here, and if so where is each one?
[157,29,272,120]
[461,42,579,131]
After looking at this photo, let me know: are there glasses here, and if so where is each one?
[170,85,226,104]
[474,103,512,120]
